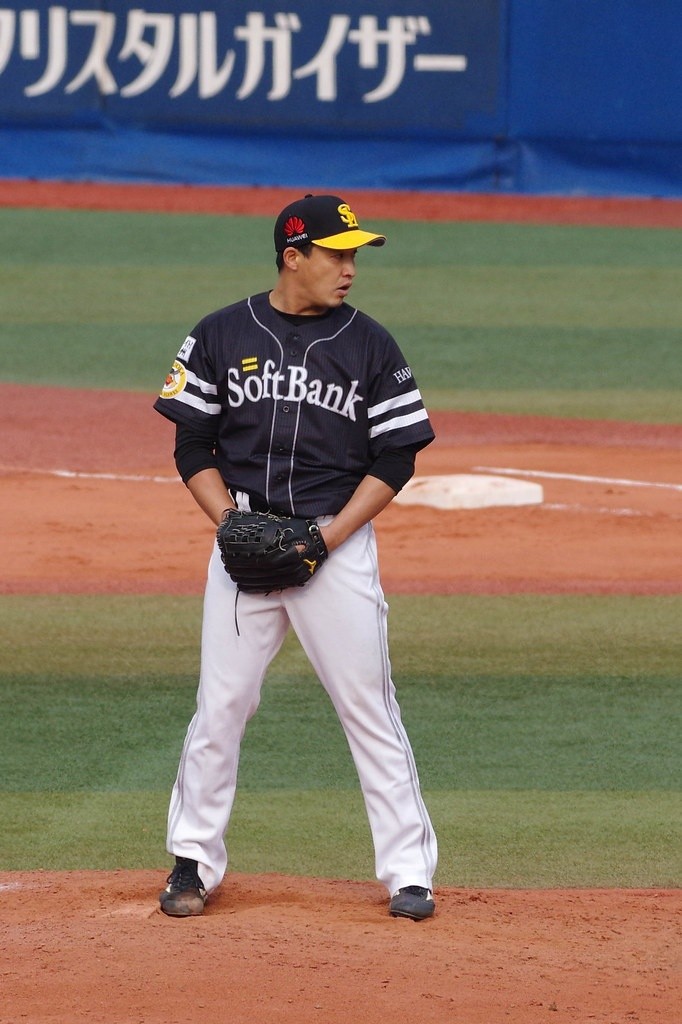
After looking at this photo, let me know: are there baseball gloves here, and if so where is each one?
[213,506,330,638]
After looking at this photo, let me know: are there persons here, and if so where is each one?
[153,195,438,919]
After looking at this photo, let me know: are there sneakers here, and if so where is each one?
[388,886,436,920]
[160,856,205,914]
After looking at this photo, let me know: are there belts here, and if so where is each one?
[230,489,295,520]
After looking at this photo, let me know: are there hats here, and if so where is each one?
[274,194,387,250]
